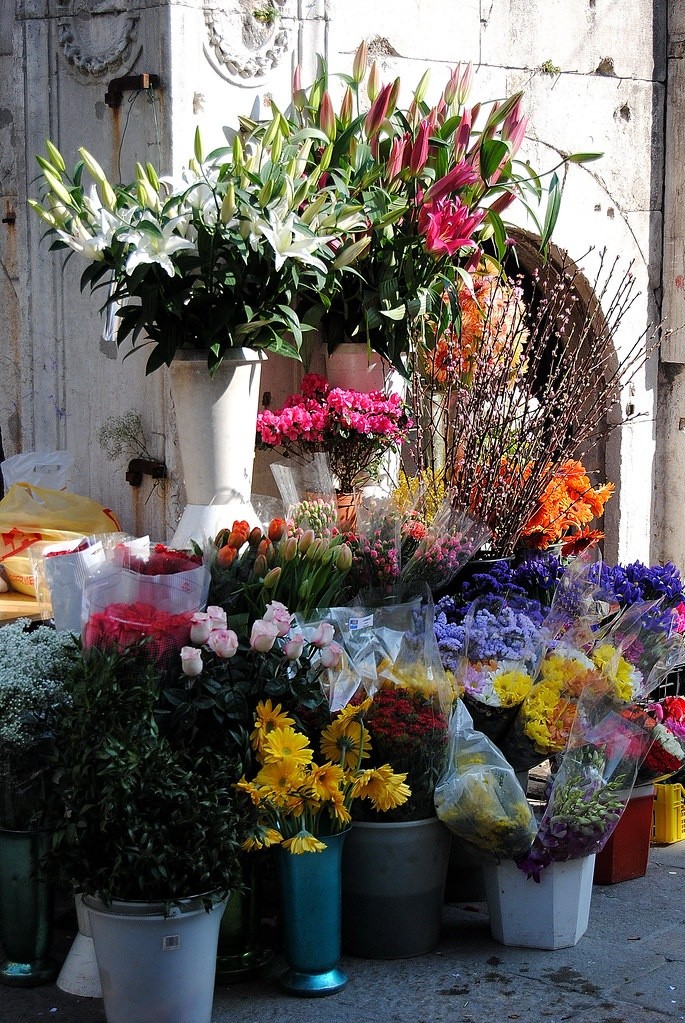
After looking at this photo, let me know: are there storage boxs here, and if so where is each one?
[650,783,685,845]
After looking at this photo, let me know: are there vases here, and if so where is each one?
[480,853,596,951]
[165,346,268,552]
[80,887,230,1023]
[321,342,407,535]
[513,769,529,797]
[593,785,655,887]
[305,487,363,533]
[432,550,515,604]
[342,817,451,960]
[260,824,350,997]
[0,828,65,988]
[57,892,103,999]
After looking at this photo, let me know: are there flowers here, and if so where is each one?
[0,36,685,921]
